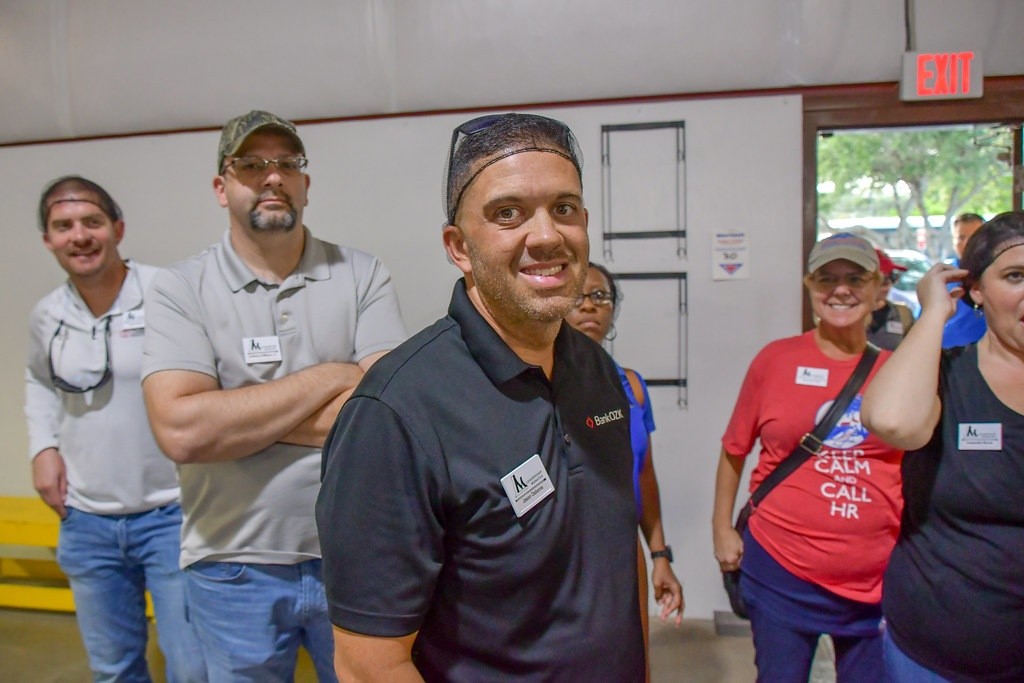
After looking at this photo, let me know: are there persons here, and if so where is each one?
[917,213,987,349]
[711,233,904,683]
[866,248,913,351]
[315,114,647,683]
[21,175,208,683]
[133,109,406,683]
[563,262,685,683]
[859,211,1024,683]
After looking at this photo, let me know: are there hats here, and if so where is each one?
[876,250,907,278]
[806,232,880,277]
[218,110,306,176]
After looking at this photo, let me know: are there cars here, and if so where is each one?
[885,250,938,320]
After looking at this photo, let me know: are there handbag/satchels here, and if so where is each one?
[723,512,749,620]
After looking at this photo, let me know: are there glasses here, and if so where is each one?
[446,113,570,225]
[572,291,615,309]
[48,315,113,392]
[220,157,308,178]
[883,273,900,283]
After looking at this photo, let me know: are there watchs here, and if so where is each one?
[651,545,673,563]
